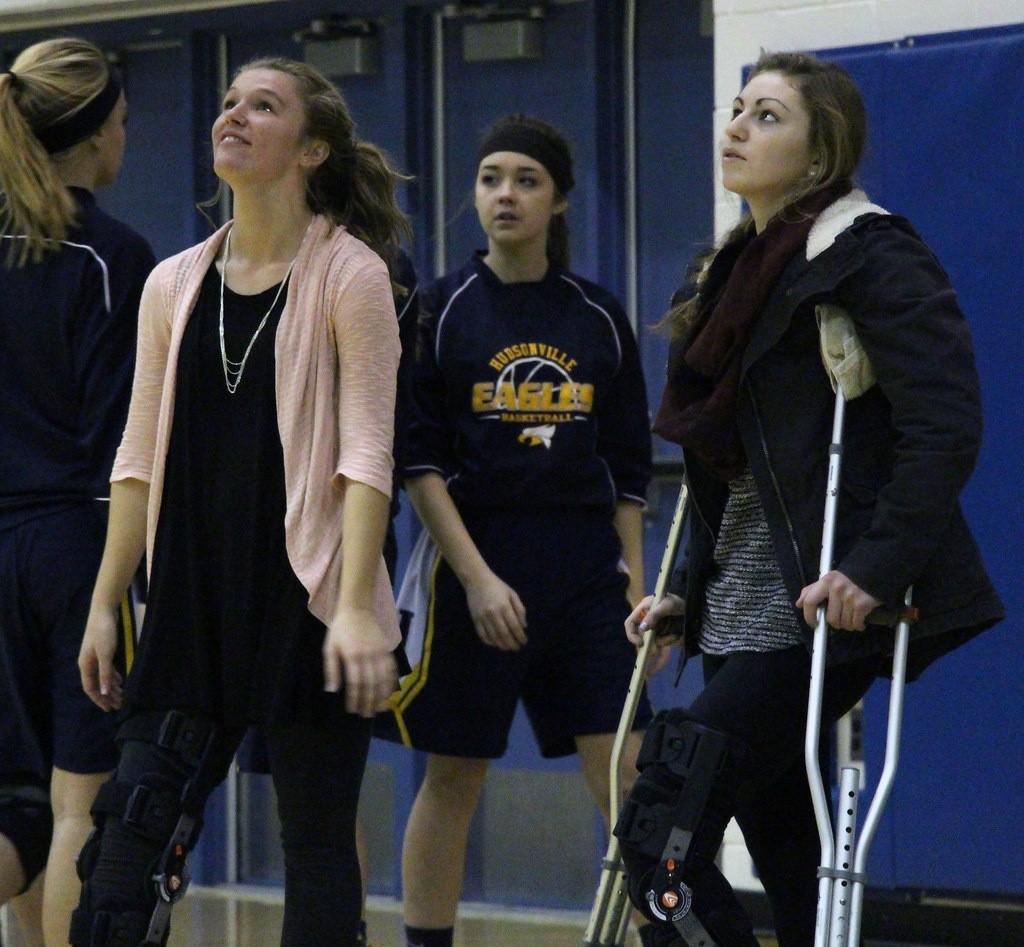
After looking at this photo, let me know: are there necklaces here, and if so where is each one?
[220,227,296,393]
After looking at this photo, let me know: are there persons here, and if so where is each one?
[0,38,159,947]
[400,121,687,947]
[615,51,1006,946]
[71,55,406,947]
